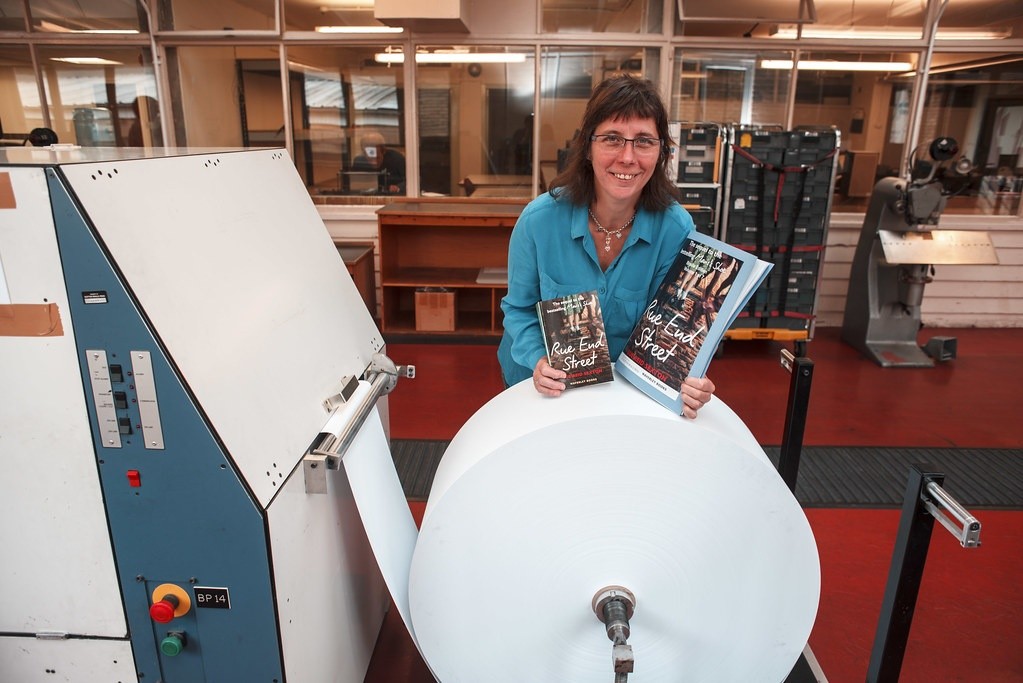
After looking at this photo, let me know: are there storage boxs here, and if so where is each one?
[414,289,457,330]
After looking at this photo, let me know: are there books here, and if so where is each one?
[534,288,615,392]
[614,229,776,418]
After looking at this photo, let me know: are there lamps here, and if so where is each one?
[374,44,525,68]
[314,24,403,33]
[768,23,1014,40]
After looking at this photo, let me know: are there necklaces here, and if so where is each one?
[587,205,639,253]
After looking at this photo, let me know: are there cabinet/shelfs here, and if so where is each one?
[840,149,880,199]
[332,240,376,319]
[374,201,527,336]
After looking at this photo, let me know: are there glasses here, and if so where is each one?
[590,135,664,153]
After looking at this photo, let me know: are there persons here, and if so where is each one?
[513,114,534,175]
[563,294,582,337]
[351,131,406,196]
[676,243,719,301]
[128,95,160,147]
[495,72,717,421]
